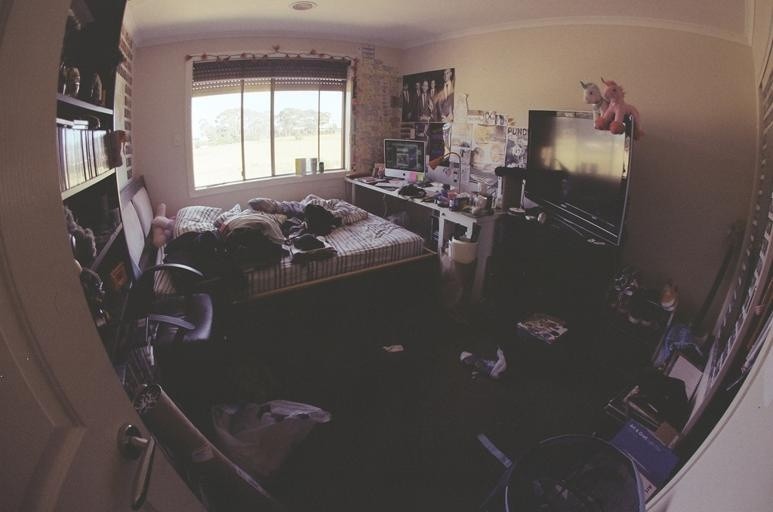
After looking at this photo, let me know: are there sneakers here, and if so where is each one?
[607,264,680,327]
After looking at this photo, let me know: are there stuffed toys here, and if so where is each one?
[596,77,644,139]
[579,81,607,118]
[150,204,175,247]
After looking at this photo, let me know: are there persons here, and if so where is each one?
[403,69,454,122]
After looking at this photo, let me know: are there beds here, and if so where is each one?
[119,175,439,298]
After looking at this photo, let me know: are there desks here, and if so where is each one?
[494,206,627,320]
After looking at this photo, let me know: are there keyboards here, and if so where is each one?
[375,182,405,189]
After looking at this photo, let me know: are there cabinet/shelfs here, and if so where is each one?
[57,0,131,384]
[345,174,501,300]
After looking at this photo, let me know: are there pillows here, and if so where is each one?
[173,205,222,239]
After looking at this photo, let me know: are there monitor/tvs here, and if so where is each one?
[524,110,634,248]
[384,138,426,184]
